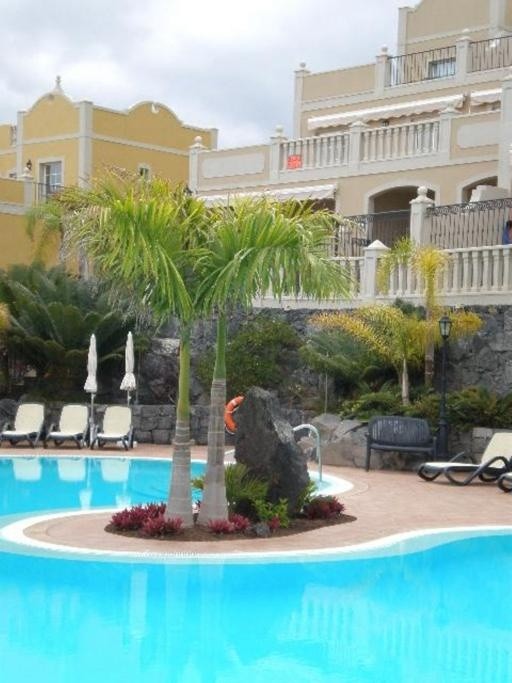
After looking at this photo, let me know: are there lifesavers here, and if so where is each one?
[224,394,245,436]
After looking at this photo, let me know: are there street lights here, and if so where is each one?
[437,311,452,461]
[178,185,192,249]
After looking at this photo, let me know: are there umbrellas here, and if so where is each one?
[120,331,137,407]
[84,333,98,439]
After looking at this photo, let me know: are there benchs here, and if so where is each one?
[364,414,438,472]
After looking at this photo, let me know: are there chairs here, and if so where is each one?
[90,405,136,451]
[417,432,512,494]
[1,402,44,448]
[44,403,90,450]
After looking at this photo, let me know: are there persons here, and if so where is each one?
[502,220,512,244]
